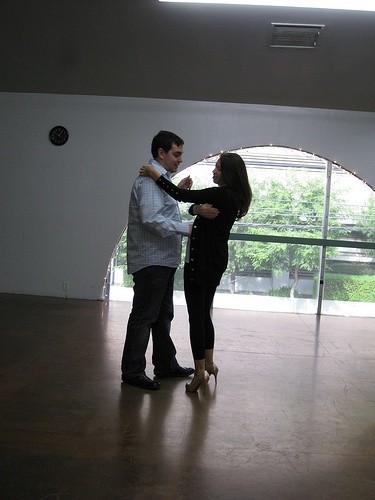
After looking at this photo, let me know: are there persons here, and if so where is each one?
[121,131,195,390]
[138,152,253,393]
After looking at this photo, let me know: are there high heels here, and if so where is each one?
[185,364,218,393]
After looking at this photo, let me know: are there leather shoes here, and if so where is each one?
[120,374,160,389]
[153,368,195,376]
